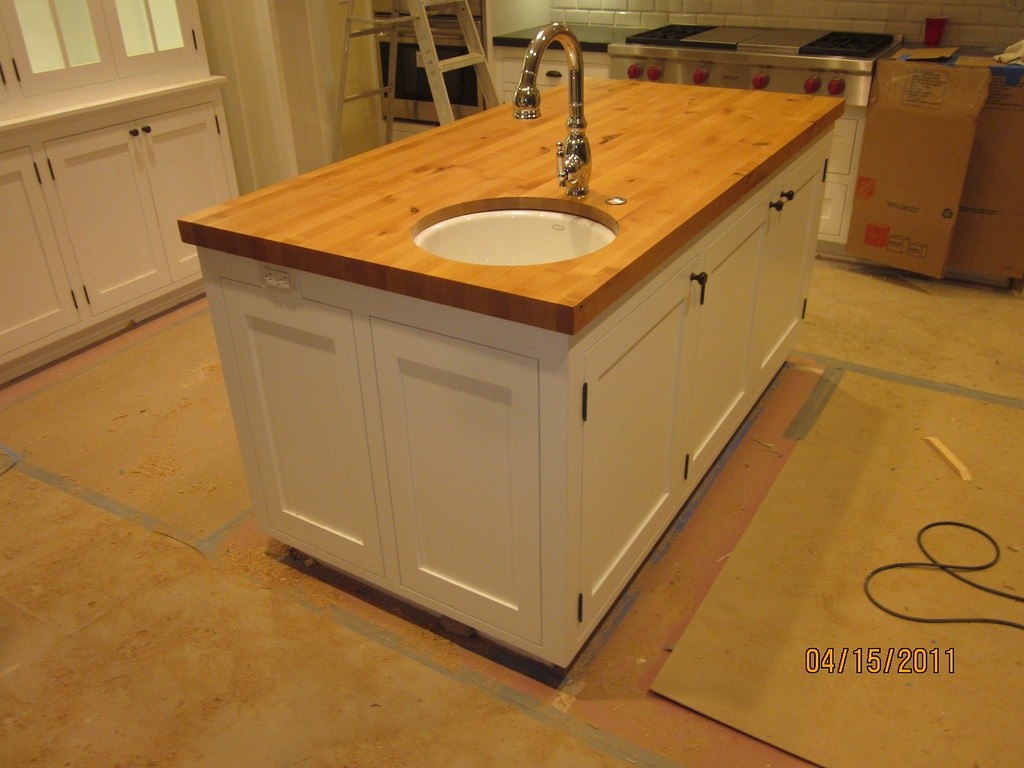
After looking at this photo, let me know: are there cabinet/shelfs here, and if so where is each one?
[577,256,701,643]
[817,115,866,245]
[683,124,834,497]
[42,102,232,317]
[503,57,609,109]
[0,146,81,359]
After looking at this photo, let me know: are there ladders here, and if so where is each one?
[330,0,503,164]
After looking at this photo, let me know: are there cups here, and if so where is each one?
[923,15,947,47]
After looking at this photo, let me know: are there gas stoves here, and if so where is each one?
[609,20,899,63]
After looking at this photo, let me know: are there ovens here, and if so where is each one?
[609,67,876,246]
